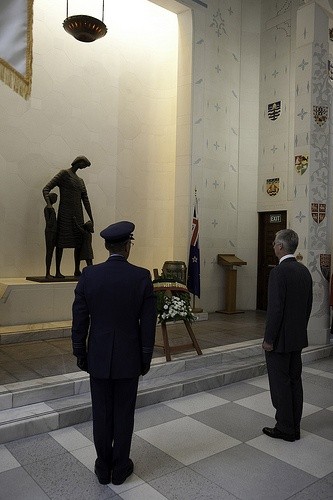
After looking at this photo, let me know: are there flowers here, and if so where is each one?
[157,296,197,325]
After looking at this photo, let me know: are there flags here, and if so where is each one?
[188,204,200,298]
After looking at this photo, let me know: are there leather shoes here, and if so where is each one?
[294,433,301,439]
[262,427,295,442]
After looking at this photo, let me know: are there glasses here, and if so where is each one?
[128,241,134,247]
[272,242,280,246]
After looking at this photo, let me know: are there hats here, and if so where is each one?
[100,221,135,242]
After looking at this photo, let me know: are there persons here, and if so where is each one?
[262,229,313,441]
[72,220,157,485]
[43,156,94,279]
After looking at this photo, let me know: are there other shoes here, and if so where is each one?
[94,458,111,485]
[111,459,134,485]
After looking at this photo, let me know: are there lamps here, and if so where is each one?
[62,0,108,43]
